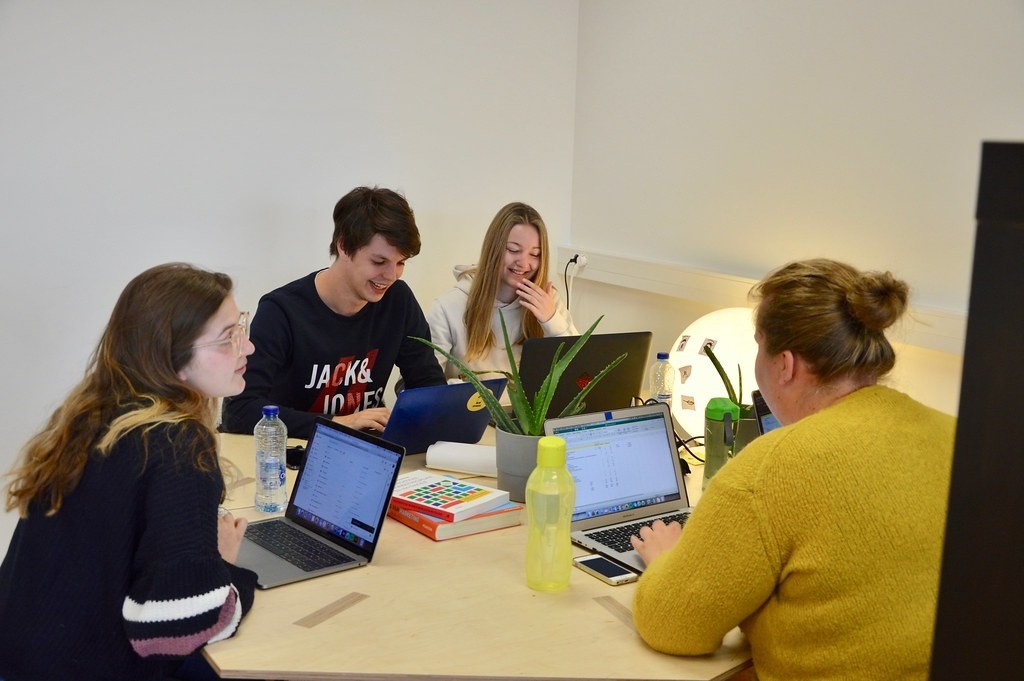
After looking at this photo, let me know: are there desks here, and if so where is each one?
[203,433,752,681]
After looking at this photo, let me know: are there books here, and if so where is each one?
[386,504,523,540]
[391,469,511,522]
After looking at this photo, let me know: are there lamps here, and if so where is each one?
[667,307,759,446]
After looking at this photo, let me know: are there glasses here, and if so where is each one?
[191,311,250,358]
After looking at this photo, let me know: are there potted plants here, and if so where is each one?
[407,307,627,503]
[704,345,761,455]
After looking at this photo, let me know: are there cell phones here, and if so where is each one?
[286,445,306,471]
[571,554,638,585]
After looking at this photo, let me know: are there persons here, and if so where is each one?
[216,187,447,442]
[426,202,580,408]
[0,262,257,681]
[630,258,958,681]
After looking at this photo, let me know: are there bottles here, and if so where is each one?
[524,436,575,592]
[649,351,675,414]
[254,404,288,514]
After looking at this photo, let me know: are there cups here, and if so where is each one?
[701,398,740,492]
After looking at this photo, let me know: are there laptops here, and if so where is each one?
[360,378,509,456]
[751,389,783,436]
[234,414,406,590]
[544,402,694,575]
[488,331,653,428]
